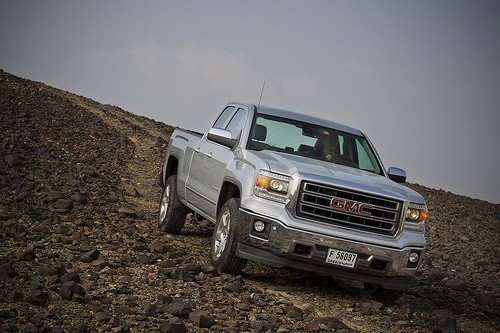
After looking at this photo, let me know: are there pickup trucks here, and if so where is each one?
[158,101,428,302]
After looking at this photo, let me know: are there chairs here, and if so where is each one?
[251,123,318,154]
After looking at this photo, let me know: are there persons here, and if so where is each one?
[309,134,341,156]
